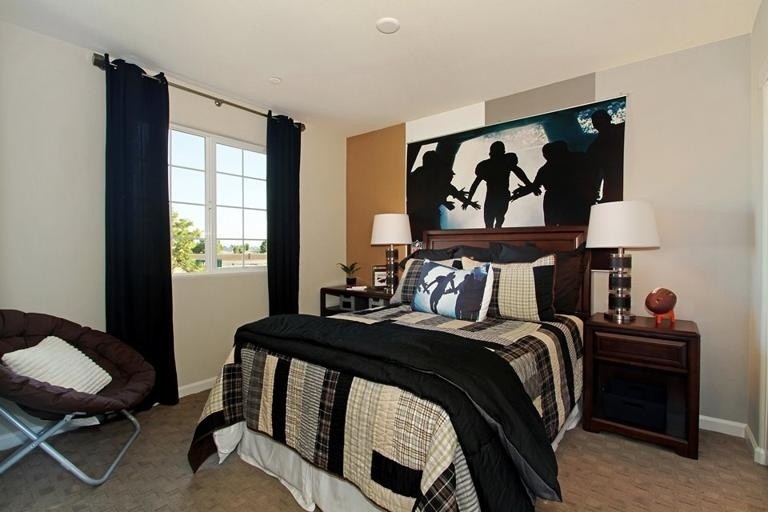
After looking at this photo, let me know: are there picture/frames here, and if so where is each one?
[371,263,389,289]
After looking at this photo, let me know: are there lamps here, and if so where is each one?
[369,213,414,296]
[583,197,661,325]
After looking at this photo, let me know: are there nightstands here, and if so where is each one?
[319,284,398,317]
[581,312,702,461]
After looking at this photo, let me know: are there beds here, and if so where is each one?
[186,226,592,512]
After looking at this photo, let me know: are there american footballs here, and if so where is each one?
[645,287,678,314]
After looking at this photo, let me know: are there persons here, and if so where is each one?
[418,268,487,322]
[407,109,626,232]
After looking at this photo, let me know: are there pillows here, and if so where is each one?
[388,241,588,324]
[2,334,113,398]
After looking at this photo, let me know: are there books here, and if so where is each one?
[351,284,370,289]
[346,288,368,292]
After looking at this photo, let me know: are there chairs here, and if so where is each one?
[1,308,157,486]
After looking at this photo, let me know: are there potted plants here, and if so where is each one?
[335,260,361,286]
